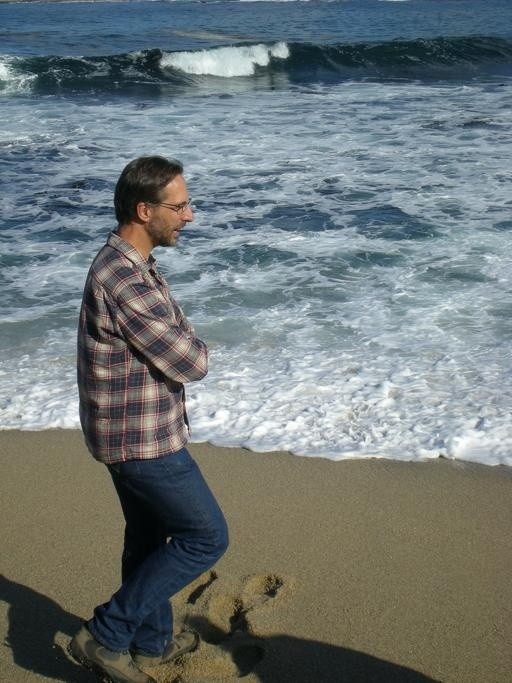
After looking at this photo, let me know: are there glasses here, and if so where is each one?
[149,197,193,213]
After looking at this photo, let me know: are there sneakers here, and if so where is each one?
[66,625,159,683]
[131,630,200,668]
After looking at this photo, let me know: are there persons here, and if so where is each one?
[50,151,229,681]
